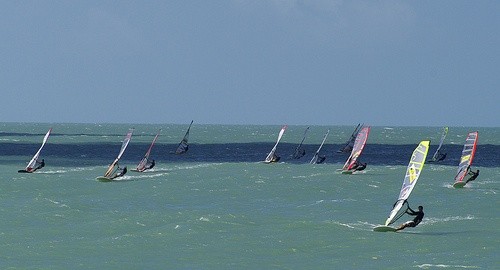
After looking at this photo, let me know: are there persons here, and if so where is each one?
[271,152,282,162]
[143,160,156,172]
[33,158,45,172]
[110,167,128,179]
[297,150,306,159]
[353,163,367,173]
[179,146,189,154]
[436,153,447,163]
[316,155,326,164]
[393,206,425,232]
[467,170,480,183]
[347,146,353,151]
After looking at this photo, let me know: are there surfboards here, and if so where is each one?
[18,170,32,173]
[373,225,396,231]
[337,169,343,171]
[131,169,140,171]
[454,181,466,188]
[428,160,435,162]
[342,170,352,175]
[97,177,110,181]
